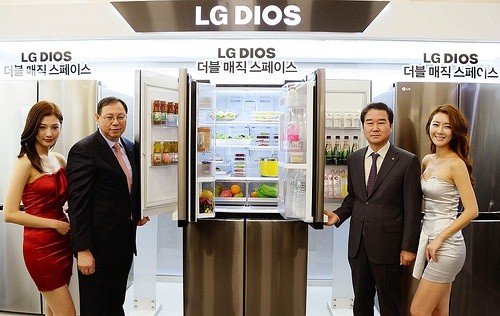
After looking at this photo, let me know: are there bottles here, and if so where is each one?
[152,139,179,166]
[153,100,179,126]
[324,169,349,198]
[334,136,341,161]
[325,136,333,160]
[342,136,350,160]
[351,135,360,152]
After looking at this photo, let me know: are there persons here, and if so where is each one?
[410,103,479,316]
[66,97,150,316]
[3,101,76,316]
[323,103,423,316]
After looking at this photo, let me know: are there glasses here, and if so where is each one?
[100,114,127,122]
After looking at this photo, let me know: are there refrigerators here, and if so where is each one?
[132,67,325,315]
[393,82,500,316]
[0,79,100,316]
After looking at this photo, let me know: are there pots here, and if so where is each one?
[255,154,279,177]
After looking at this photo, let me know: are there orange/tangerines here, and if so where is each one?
[231,185,240,194]
[201,190,213,199]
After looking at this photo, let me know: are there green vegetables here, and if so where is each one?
[257,183,277,198]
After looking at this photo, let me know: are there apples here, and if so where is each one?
[220,190,232,197]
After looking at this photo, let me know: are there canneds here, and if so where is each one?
[197,126,211,151]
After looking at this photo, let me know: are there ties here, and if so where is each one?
[367,154,380,196]
[112,142,132,192]
